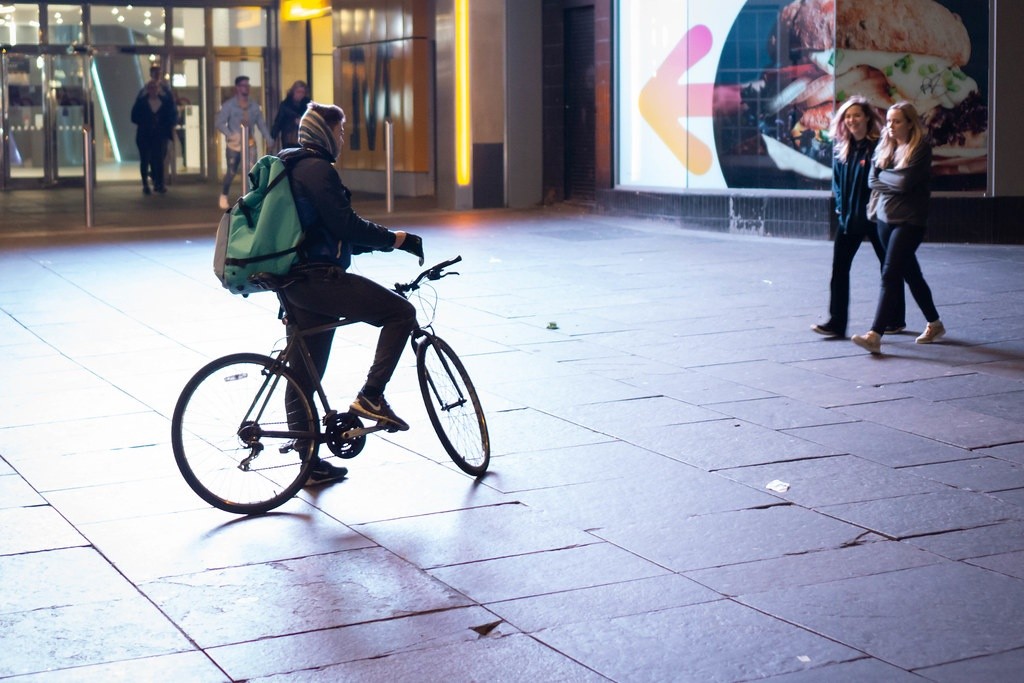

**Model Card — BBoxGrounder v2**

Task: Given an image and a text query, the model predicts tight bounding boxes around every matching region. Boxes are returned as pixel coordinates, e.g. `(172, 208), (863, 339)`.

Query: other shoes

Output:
(142, 185), (167, 195)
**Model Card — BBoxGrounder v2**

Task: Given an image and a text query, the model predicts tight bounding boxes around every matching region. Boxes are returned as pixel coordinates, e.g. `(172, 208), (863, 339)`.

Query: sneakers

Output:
(810, 317), (846, 339)
(300, 460), (348, 486)
(219, 194), (229, 209)
(851, 330), (881, 354)
(350, 392), (409, 432)
(916, 321), (946, 343)
(884, 323), (906, 334)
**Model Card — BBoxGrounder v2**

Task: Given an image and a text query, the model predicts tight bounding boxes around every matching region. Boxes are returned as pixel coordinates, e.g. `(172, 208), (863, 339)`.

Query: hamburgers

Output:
(742, 0), (991, 177)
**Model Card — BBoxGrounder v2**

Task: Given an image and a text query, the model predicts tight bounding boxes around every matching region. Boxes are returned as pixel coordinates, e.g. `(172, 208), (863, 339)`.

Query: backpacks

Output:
(213, 153), (333, 298)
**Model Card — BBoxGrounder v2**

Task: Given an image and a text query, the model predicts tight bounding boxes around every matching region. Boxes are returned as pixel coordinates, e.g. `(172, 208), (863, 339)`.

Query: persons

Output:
(130, 66), (180, 197)
(214, 76), (275, 209)
(270, 79), (312, 150)
(809, 96), (906, 337)
(849, 99), (947, 354)
(268, 102), (425, 489)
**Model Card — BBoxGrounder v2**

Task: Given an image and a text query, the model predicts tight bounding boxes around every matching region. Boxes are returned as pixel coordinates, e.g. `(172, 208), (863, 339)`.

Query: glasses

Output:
(237, 84), (251, 88)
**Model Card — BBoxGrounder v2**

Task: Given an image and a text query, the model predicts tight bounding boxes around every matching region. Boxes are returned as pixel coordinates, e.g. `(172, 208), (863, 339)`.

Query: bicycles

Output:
(170, 253), (492, 516)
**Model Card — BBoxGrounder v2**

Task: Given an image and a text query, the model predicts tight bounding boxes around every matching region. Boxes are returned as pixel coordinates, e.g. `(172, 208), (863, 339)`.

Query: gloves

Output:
(398, 233), (424, 267)
